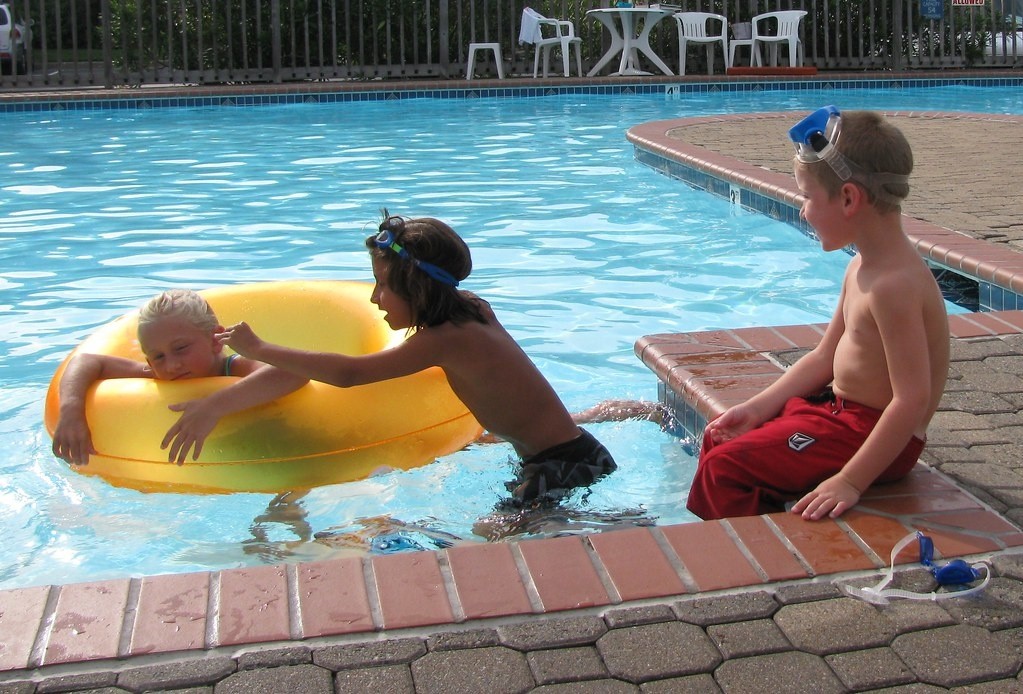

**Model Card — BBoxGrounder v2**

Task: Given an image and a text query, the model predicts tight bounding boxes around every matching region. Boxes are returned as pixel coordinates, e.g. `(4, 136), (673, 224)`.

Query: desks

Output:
(585, 8), (676, 76)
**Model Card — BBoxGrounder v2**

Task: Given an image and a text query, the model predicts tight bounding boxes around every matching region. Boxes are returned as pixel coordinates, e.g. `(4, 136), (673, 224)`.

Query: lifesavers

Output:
(45, 280), (483, 495)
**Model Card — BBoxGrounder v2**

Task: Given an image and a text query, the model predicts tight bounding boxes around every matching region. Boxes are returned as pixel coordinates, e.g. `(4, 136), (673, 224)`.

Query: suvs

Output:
(0, 3), (36, 77)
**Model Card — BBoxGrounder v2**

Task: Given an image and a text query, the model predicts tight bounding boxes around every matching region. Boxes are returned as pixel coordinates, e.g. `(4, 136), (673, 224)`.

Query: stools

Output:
(467, 43), (505, 79)
(728, 40), (762, 67)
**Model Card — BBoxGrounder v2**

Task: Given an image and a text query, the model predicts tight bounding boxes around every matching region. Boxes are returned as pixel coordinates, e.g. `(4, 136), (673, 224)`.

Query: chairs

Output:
(750, 10), (808, 68)
(672, 12), (729, 76)
(524, 7), (582, 78)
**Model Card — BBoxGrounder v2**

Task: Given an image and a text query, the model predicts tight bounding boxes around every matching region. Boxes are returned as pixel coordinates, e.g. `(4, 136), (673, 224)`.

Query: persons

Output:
(686, 105), (950, 523)
(50, 289), (311, 465)
(214, 208), (617, 501)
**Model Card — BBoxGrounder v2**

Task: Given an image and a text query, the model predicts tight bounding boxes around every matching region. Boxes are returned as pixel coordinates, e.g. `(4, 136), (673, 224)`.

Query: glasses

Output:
(919, 535), (978, 586)
(788, 105), (842, 164)
(374, 228), (394, 248)
